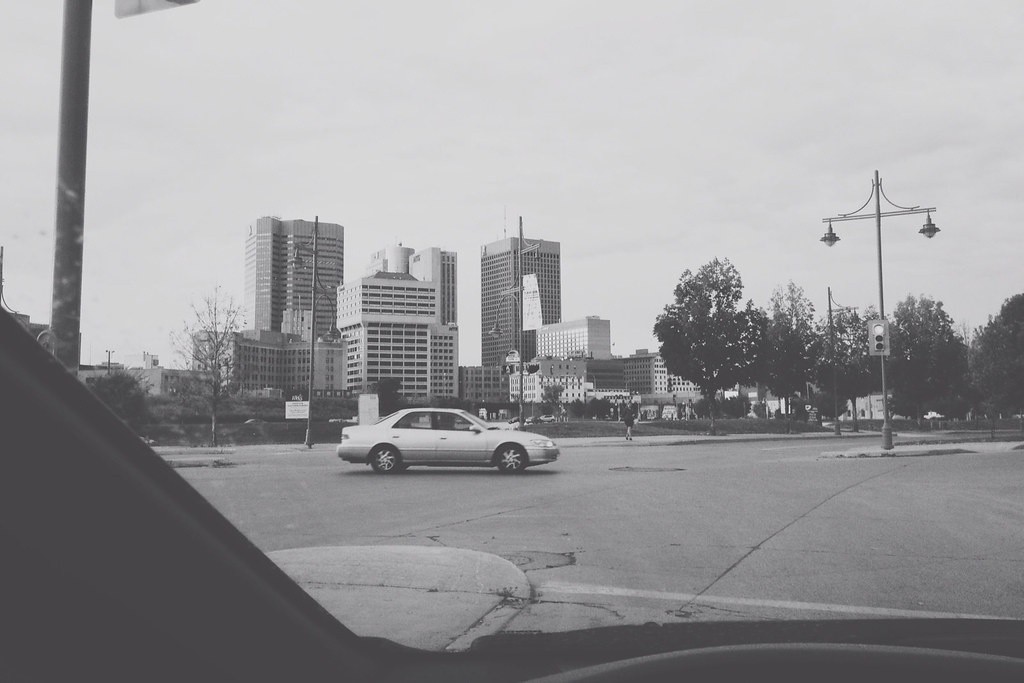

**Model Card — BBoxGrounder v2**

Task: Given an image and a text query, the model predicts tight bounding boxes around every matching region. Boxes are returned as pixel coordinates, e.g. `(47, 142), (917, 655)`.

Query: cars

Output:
(245, 419), (264, 423)
(508, 415), (526, 424)
(335, 406), (561, 476)
(525, 416), (541, 424)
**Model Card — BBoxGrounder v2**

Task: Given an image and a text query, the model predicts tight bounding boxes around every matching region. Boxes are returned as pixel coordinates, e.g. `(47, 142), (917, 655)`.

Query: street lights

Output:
(288, 216), (343, 449)
(826, 286), (859, 436)
(106, 350), (115, 375)
(487, 216), (541, 422)
(819, 169), (941, 450)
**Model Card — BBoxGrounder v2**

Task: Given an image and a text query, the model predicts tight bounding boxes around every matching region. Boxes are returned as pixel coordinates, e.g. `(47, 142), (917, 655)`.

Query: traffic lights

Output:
(867, 320), (890, 356)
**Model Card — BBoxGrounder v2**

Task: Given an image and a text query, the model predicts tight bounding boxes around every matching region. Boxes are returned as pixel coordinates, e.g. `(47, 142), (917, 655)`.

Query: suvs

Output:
(540, 414), (556, 423)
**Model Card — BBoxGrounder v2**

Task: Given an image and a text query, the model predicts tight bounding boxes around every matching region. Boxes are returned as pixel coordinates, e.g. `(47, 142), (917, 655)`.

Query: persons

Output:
(622, 403), (635, 440)
